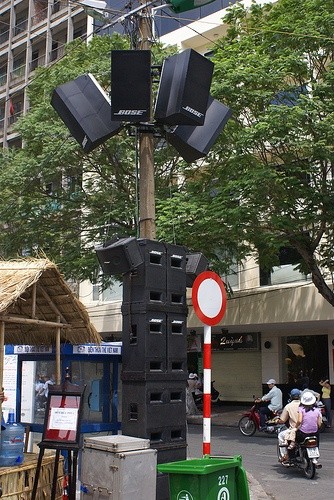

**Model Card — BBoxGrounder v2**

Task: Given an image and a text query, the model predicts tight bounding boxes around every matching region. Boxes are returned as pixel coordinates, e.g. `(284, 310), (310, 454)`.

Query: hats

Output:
(267, 378), (276, 384)
(303, 384), (309, 389)
(189, 373), (195, 378)
(301, 392), (316, 405)
(196, 381), (202, 386)
(291, 388), (301, 395)
(194, 374), (198, 377)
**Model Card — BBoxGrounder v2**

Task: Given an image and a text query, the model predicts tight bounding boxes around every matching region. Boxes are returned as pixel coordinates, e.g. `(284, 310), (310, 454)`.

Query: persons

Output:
(255, 376), (333, 461)
(185, 372), (204, 416)
(34, 374), (55, 414)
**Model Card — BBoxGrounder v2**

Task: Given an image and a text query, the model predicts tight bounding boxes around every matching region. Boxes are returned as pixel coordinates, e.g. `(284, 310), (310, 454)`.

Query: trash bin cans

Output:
(157, 454), (249, 500)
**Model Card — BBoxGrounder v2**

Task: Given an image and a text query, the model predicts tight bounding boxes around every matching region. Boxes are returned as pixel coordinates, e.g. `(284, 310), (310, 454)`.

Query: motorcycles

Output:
(274, 416), (322, 480)
(238, 395), (289, 438)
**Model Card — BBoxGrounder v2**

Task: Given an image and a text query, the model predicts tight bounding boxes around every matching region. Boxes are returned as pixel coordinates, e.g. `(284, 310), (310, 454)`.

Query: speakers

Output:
(153, 48), (214, 126)
(111, 50), (153, 123)
(50, 73), (126, 154)
(185, 252), (209, 288)
(168, 94), (231, 163)
(94, 236), (144, 274)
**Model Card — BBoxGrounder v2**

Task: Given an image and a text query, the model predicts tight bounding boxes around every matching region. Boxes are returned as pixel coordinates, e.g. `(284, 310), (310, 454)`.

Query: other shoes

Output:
(287, 443), (295, 450)
(279, 455), (290, 462)
(279, 442), (288, 447)
(289, 459), (295, 466)
(259, 426), (267, 430)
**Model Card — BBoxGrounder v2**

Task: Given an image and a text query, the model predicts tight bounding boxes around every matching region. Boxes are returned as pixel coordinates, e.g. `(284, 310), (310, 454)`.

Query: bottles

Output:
(0, 410), (25, 467)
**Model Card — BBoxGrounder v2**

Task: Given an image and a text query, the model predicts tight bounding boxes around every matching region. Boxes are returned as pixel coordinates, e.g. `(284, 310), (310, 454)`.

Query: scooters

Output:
(194, 380), (222, 406)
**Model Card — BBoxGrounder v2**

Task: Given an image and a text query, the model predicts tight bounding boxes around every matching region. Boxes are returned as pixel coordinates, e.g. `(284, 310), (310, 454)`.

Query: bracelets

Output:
(277, 420), (280, 424)
